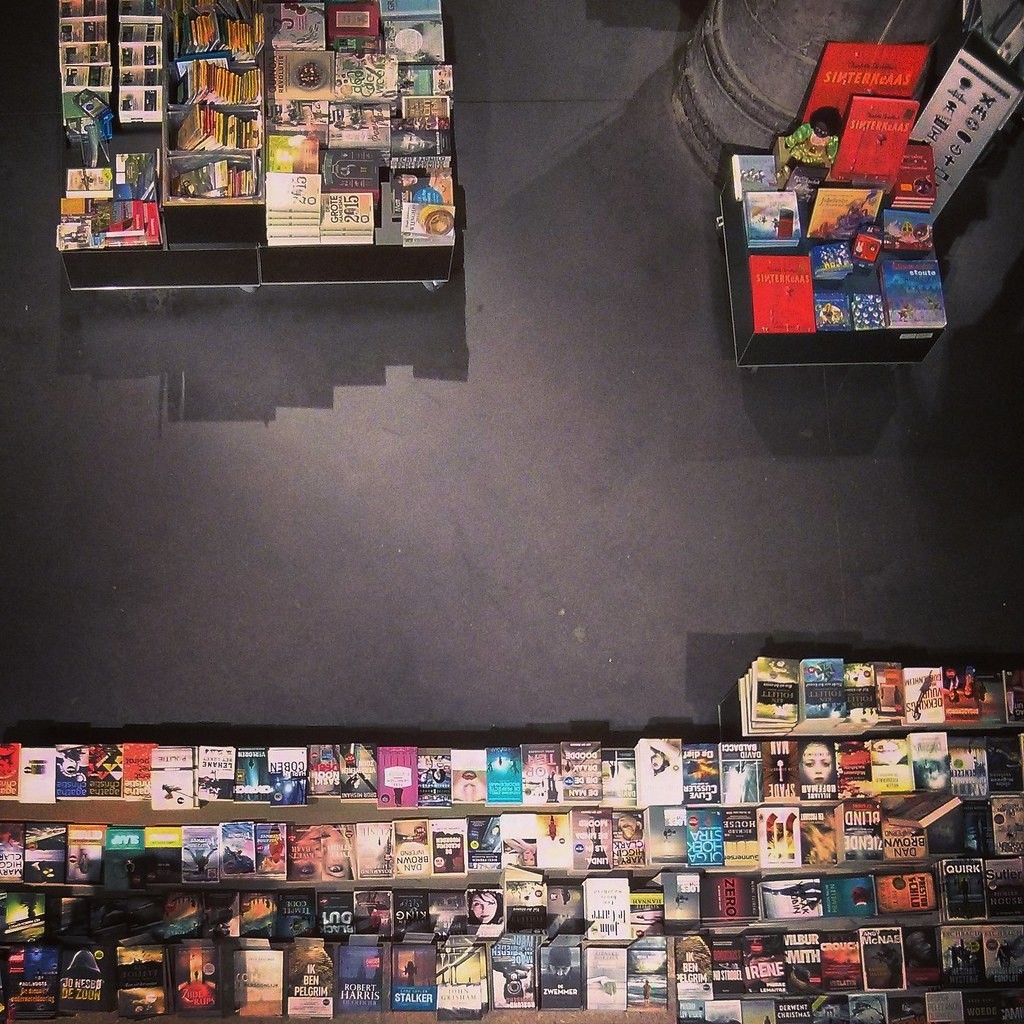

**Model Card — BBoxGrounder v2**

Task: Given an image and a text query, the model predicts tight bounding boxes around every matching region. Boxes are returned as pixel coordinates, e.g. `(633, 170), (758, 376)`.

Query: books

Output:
(56, 1), (457, 251)
(731, 96), (947, 334)
(0, 655), (1024, 1024)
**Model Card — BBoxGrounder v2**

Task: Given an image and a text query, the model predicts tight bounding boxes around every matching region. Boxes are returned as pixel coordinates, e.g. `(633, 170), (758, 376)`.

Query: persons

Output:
(880, 683), (885, 701)
(798, 741), (838, 799)
(392, 171), (436, 193)
(69, 844), (91, 881)
(391, 130), (439, 157)
(648, 739), (681, 776)
(905, 931), (936, 985)
(291, 825), (357, 882)
(467, 890), (503, 925)
(644, 979), (651, 1008)
(894, 686), (902, 711)
(961, 664), (981, 698)
(493, 961), (531, 1004)
(370, 908), (380, 932)
(270, 18), (294, 40)
(540, 947), (581, 993)
(617, 811), (644, 842)
(775, 107), (838, 182)
(995, 939), (1017, 970)
(943, 668), (960, 702)
(505, 837), (537, 868)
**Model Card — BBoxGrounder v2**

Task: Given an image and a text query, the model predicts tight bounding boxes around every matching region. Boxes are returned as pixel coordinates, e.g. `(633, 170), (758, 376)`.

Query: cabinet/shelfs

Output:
(55, 0), (467, 294)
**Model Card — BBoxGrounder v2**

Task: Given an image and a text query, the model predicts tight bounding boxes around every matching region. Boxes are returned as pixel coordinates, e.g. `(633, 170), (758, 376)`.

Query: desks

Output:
(715, 170), (949, 369)
